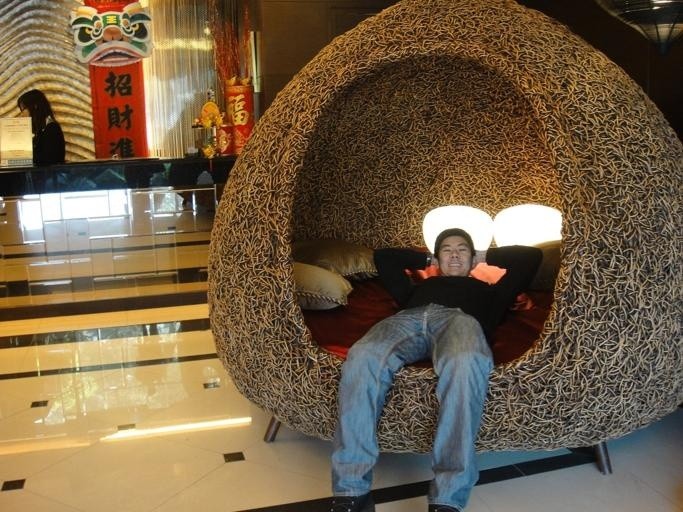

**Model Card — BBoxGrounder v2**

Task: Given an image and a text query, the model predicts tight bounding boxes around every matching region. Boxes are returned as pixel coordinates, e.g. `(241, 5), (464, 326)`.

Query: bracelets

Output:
(426, 251), (433, 270)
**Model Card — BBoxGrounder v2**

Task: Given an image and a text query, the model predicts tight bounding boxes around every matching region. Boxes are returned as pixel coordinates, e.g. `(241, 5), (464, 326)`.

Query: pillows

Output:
(521, 239), (564, 294)
(291, 237), (378, 310)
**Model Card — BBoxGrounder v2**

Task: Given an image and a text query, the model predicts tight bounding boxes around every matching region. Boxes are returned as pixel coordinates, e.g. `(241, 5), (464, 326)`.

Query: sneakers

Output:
(328, 488), (375, 512)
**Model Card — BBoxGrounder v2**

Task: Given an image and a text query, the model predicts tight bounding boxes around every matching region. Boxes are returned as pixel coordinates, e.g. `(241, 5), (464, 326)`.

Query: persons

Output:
(328, 229), (545, 512)
(16, 90), (63, 166)
